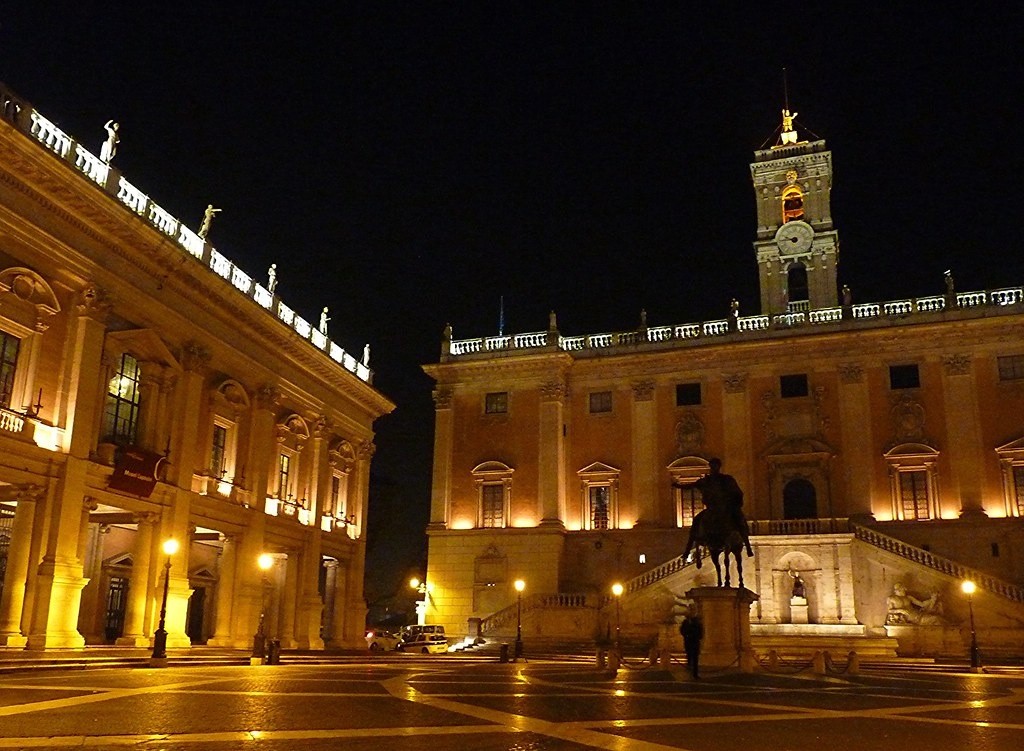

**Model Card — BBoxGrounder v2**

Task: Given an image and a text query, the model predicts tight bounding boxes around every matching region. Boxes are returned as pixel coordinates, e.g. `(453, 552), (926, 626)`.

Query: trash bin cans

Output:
(269, 638), (281, 665)
(500, 643), (510, 663)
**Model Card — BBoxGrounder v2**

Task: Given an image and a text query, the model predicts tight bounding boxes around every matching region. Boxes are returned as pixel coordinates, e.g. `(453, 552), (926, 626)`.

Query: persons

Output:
(548, 310), (559, 332)
(103, 118), (121, 165)
(364, 344), (370, 368)
(266, 261), (278, 296)
(319, 307), (331, 336)
(842, 284), (852, 306)
(640, 308), (647, 326)
(781, 108), (798, 133)
(787, 570), (805, 598)
(443, 321), (455, 345)
(196, 203), (222, 240)
(671, 458), (760, 560)
(729, 296), (740, 318)
(945, 271), (955, 295)
(885, 580), (953, 626)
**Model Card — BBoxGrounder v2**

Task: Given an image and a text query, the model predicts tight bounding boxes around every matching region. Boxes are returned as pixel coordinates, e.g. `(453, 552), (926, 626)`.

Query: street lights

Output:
(150, 539), (178, 667)
(250, 555), (273, 666)
(962, 581), (983, 674)
(513, 580), (527, 663)
(612, 584), (623, 641)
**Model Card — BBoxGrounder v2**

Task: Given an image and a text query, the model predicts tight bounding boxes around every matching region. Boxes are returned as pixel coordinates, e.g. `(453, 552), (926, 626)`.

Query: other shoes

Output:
(692, 673), (699, 679)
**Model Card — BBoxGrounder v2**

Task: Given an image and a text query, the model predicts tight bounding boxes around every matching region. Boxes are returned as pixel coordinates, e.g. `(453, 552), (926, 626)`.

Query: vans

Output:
(392, 624), (445, 642)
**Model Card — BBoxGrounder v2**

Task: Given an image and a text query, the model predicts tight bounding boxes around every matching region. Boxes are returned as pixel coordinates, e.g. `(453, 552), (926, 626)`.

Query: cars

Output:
(400, 633), (448, 654)
(364, 630), (402, 651)
